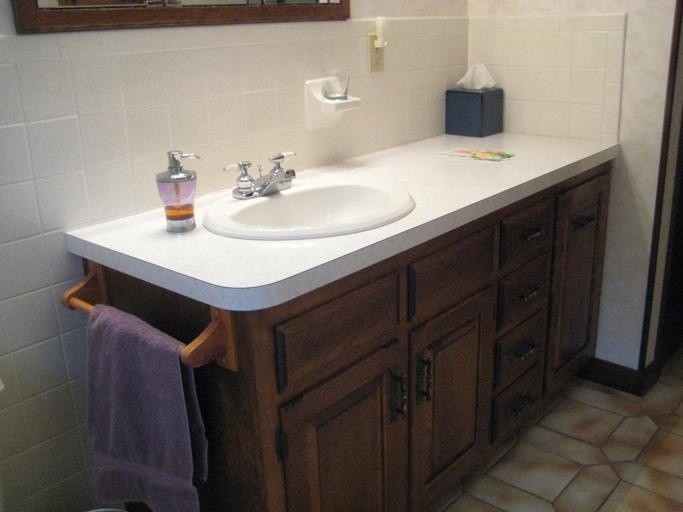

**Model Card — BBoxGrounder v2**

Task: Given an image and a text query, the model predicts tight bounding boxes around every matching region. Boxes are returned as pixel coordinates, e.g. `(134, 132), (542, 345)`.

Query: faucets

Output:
(258, 166), (297, 196)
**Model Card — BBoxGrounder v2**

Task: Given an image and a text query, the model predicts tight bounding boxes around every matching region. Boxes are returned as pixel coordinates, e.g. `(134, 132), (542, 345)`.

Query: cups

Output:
(321, 70), (349, 100)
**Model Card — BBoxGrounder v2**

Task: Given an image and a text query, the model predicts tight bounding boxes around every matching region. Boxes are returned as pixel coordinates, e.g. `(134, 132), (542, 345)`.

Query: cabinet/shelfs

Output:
(62, 195), (496, 512)
(493, 173), (555, 464)
(547, 150), (610, 402)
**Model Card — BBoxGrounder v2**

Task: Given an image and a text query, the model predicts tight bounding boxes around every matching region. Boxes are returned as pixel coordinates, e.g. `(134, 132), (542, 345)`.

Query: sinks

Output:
(203, 176), (418, 240)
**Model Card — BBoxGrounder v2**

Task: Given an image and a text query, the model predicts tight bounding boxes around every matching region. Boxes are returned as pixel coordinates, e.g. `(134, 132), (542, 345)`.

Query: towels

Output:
(87, 303), (207, 512)
